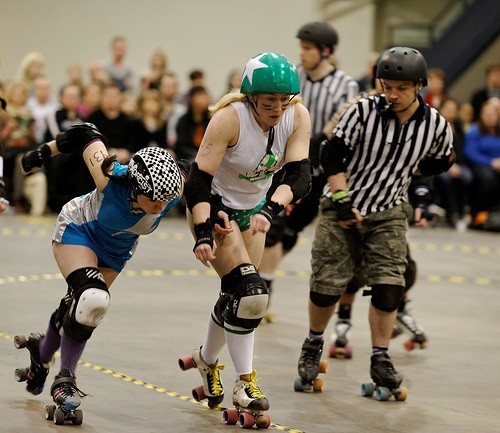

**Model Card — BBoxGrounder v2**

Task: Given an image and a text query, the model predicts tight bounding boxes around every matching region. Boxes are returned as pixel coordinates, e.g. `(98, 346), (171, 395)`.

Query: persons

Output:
(295, 46), (456, 402)
(177, 49), (312, 428)
(315, 79), (427, 358)
(13, 123), (185, 425)
(0, 34), (500, 231)
(244, 21), (359, 321)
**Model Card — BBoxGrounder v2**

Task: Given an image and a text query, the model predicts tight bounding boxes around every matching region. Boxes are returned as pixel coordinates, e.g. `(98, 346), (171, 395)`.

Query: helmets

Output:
(376, 46), (428, 87)
(128, 146), (182, 202)
(297, 22), (338, 46)
(239, 52), (301, 94)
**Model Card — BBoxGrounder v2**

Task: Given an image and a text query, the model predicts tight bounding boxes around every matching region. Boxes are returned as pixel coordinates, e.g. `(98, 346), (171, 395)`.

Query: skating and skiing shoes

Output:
(178, 345), (225, 404)
(360, 351), (408, 401)
(391, 312), (428, 350)
(14, 332), (55, 396)
(294, 337), (328, 392)
(223, 369), (271, 429)
(45, 368), (87, 425)
(328, 321), (352, 358)
